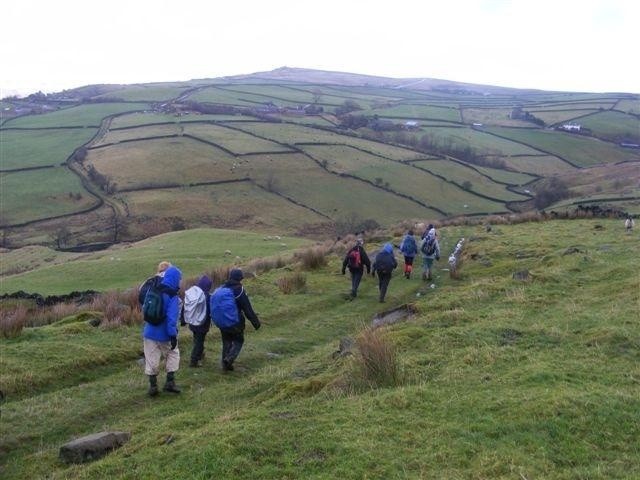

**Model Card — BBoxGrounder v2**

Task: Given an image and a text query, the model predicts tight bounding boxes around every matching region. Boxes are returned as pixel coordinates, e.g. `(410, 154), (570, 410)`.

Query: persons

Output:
(142, 267), (182, 395)
(419, 228), (440, 281)
(217, 268), (261, 373)
(180, 274), (215, 366)
(398, 230), (420, 279)
(623, 214), (635, 235)
(342, 236), (371, 298)
(137, 261), (174, 306)
(371, 242), (397, 302)
(421, 222), (437, 241)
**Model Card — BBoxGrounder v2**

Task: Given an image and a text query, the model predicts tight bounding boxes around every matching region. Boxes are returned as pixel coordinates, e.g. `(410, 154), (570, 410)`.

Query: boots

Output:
(404, 271), (433, 280)
(148, 353), (237, 396)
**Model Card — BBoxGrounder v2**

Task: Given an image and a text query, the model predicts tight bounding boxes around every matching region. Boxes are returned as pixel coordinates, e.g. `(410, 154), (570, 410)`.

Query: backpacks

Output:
(142, 287), (164, 326)
(376, 231), (437, 274)
(209, 287), (240, 329)
(348, 250), (361, 268)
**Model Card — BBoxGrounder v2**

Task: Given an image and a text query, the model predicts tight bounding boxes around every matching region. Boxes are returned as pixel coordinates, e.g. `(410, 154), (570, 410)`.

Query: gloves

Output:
(183, 284), (207, 326)
(170, 336), (177, 350)
